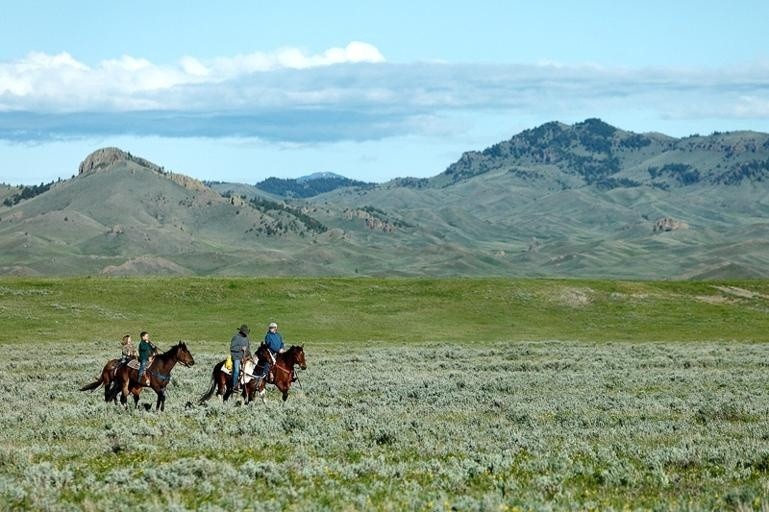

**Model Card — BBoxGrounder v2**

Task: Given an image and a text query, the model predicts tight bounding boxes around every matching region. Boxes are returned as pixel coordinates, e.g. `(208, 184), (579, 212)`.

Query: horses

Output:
(198, 341), (271, 405)
(78, 345), (158, 405)
(106, 340), (195, 412)
(241, 343), (307, 401)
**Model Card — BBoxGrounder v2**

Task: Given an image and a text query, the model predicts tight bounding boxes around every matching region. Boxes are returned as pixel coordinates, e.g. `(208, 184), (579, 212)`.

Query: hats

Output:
(269, 323), (277, 328)
(236, 324), (250, 335)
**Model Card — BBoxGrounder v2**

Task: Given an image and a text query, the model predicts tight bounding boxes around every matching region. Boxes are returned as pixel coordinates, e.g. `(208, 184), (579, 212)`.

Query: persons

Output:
(114, 334), (139, 387)
(259, 322), (286, 380)
(230, 323), (252, 393)
(135, 331), (158, 391)
